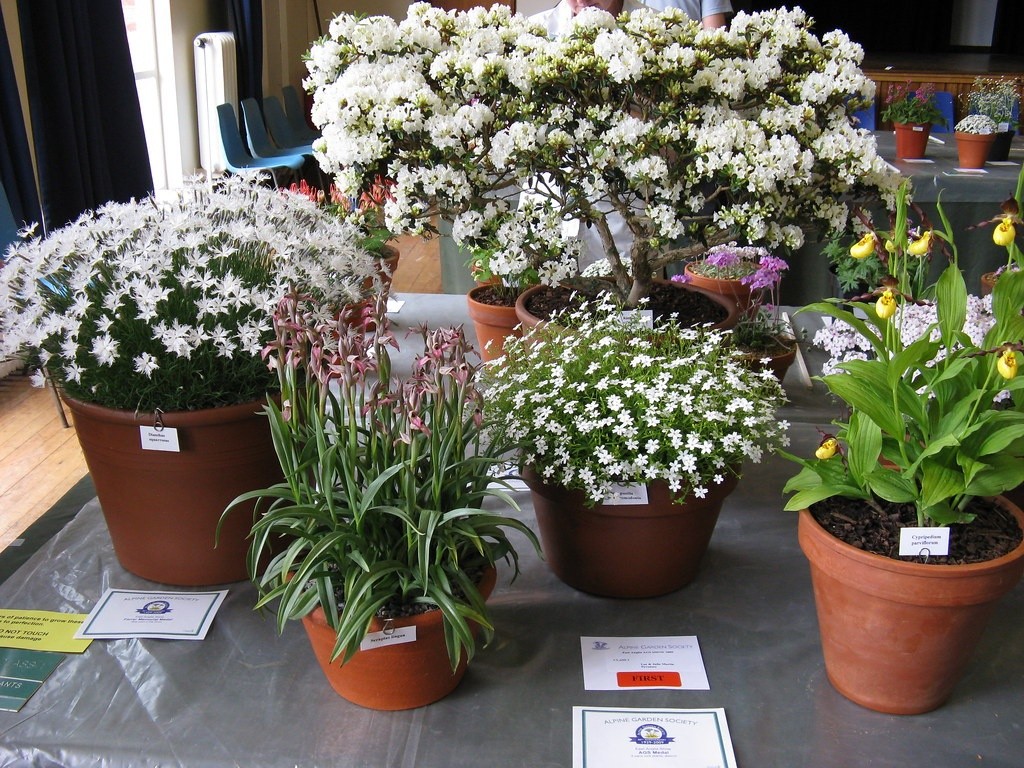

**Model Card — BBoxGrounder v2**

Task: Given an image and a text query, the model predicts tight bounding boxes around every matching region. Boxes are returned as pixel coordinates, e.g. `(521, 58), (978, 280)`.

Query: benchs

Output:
(217, 88), (323, 185)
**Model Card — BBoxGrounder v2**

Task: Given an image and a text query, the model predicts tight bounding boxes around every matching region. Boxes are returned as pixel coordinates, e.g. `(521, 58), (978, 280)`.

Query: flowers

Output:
(958, 76), (1021, 131)
(772, 178), (1024, 528)
(287, 179), (394, 260)
(302, 5), (903, 307)
(0, 167), (390, 412)
(215, 287), (545, 674)
(955, 114), (997, 136)
(708, 252), (805, 353)
(882, 81), (950, 131)
(475, 292), (791, 505)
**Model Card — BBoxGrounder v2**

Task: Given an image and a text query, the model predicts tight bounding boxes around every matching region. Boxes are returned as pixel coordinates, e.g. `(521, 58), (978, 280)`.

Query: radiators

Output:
(192, 30), (240, 180)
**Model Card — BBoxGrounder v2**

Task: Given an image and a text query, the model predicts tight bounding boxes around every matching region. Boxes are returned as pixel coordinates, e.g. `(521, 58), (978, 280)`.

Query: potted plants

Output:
(466, 259), (525, 364)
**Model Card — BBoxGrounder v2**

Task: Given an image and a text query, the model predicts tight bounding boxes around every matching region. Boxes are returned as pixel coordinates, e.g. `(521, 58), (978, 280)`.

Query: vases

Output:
(320, 303), (367, 377)
(681, 261), (767, 331)
(894, 122), (931, 158)
(987, 130), (1015, 162)
(954, 131), (997, 169)
(354, 247), (400, 329)
(798, 472), (1024, 716)
(518, 450), (740, 601)
(285, 559), (495, 712)
(727, 338), (796, 384)
(57, 378), (324, 588)
(515, 277), (739, 356)
